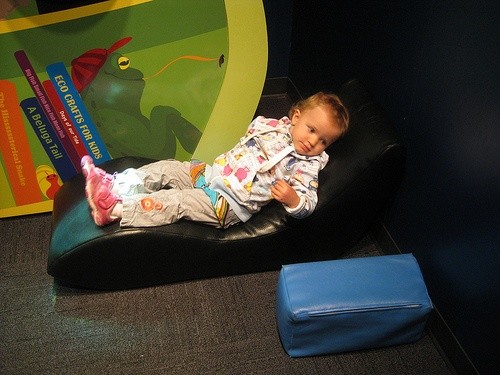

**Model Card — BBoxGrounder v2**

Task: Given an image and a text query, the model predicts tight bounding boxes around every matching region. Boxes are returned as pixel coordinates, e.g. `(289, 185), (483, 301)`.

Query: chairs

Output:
(47, 97), (408, 292)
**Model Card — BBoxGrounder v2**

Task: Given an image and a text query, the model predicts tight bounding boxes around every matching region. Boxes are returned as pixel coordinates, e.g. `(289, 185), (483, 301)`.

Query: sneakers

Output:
(81, 155), (113, 189)
(85, 172), (120, 226)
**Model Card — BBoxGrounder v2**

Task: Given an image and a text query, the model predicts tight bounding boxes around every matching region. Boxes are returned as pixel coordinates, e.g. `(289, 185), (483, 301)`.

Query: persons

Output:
(81, 92), (350, 228)
